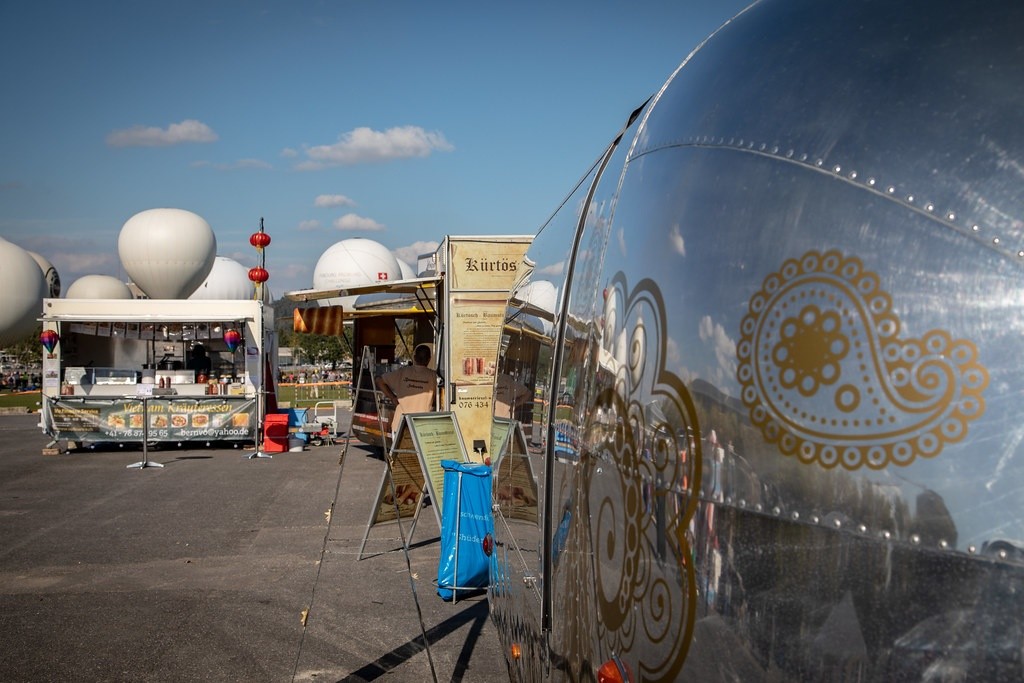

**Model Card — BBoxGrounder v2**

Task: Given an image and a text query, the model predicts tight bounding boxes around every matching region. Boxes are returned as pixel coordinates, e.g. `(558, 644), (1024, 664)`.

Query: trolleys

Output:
(310, 402), (337, 446)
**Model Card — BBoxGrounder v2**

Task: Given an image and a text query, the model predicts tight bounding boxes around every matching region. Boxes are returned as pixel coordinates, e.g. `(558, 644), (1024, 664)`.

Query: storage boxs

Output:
(263, 413), (289, 453)
(42, 448), (60, 456)
(288, 439), (304, 453)
(278, 402), (290, 409)
(244, 445), (260, 450)
(152, 388), (175, 395)
(277, 407), (309, 445)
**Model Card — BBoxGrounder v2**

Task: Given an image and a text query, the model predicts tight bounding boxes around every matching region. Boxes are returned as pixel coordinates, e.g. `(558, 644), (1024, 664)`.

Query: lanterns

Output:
(249, 217), (271, 300)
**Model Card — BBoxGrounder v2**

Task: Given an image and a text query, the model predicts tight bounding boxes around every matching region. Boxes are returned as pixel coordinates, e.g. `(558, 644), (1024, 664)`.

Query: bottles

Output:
(207, 373), (218, 385)
(219, 374), (233, 384)
(208, 382), (218, 395)
(159, 378), (164, 388)
(220, 384), (226, 395)
(60, 382), (67, 395)
(166, 377), (170, 388)
(197, 371), (207, 383)
(66, 385), (74, 395)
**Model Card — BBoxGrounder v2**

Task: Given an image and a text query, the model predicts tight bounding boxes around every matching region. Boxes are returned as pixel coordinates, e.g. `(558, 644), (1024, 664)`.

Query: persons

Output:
(186, 345), (211, 383)
(375, 345), (437, 443)
(277, 370), (353, 398)
(0, 371), (41, 392)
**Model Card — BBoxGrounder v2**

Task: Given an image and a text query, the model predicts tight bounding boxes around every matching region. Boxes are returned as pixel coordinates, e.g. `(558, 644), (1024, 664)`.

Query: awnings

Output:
(36, 315), (254, 325)
(276, 276), (444, 320)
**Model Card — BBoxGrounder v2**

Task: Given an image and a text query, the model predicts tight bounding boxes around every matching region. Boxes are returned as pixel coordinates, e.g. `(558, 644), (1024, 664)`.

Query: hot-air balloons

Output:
(223, 330), (241, 353)
(118, 208), (216, 299)
(314, 239), (419, 372)
(40, 330), (58, 358)
(0, 237), (61, 387)
(65, 274), (133, 299)
(187, 257), (274, 315)
